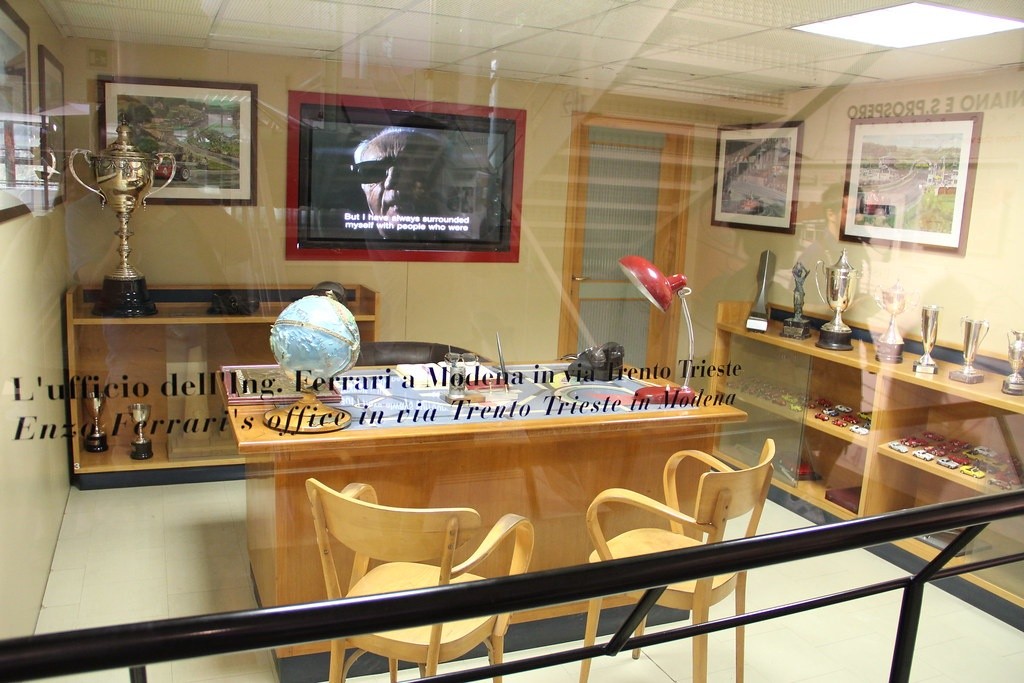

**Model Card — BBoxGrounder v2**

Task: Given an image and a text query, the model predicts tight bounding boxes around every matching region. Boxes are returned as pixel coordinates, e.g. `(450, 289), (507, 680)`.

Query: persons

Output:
(325, 126), (469, 240)
(794, 182), (874, 488)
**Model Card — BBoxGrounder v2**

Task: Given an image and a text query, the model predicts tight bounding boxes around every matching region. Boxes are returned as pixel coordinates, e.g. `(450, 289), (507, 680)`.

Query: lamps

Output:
(619, 256), (696, 405)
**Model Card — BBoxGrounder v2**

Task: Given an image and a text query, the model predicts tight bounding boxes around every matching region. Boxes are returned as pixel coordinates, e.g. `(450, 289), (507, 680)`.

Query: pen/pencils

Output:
(495, 330), (510, 394)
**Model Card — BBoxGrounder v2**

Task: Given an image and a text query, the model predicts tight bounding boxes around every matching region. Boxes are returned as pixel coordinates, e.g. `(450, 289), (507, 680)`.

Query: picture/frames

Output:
(839, 111), (984, 257)
(38, 44), (67, 210)
(0, 0), (35, 222)
(220, 365), (342, 405)
(711, 120), (805, 234)
(97, 75), (258, 206)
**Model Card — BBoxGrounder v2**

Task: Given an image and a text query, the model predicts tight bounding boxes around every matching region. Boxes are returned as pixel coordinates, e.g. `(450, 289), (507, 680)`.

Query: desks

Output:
(214, 362), (747, 683)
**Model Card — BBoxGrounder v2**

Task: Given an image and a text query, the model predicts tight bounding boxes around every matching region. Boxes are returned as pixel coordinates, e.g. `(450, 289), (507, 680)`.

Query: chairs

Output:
(305, 478), (535, 683)
(579, 438), (776, 683)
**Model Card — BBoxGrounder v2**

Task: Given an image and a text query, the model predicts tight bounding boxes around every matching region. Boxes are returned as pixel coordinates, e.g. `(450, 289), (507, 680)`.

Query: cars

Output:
(153, 161), (190, 181)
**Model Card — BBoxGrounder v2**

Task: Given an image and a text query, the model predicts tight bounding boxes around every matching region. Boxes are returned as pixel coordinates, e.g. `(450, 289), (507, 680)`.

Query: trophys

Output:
(127, 401), (155, 460)
(82, 391), (109, 452)
(780, 260), (813, 341)
(813, 247), (870, 352)
(948, 316), (988, 383)
(874, 277), (919, 364)
(1002, 328), (1024, 395)
(741, 250), (770, 333)
(912, 304), (944, 375)
(68, 114), (177, 317)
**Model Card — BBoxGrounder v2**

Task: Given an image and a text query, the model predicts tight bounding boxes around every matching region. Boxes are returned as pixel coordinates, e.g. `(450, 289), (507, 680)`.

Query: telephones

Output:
(567, 342), (625, 381)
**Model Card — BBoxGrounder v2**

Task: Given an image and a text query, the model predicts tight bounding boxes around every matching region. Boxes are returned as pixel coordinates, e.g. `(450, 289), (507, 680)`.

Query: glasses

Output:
(348, 160), (433, 183)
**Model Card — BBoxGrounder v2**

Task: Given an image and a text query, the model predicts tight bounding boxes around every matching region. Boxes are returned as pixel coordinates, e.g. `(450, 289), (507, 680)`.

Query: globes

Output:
(263, 281), (360, 434)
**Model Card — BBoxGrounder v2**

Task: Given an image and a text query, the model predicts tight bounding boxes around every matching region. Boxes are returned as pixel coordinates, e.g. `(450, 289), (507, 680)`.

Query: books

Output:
(395, 360), (504, 389)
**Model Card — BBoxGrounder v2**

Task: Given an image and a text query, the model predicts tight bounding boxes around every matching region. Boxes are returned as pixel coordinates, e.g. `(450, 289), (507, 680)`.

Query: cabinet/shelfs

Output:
(708, 300), (1024, 608)
(66, 284), (381, 490)
